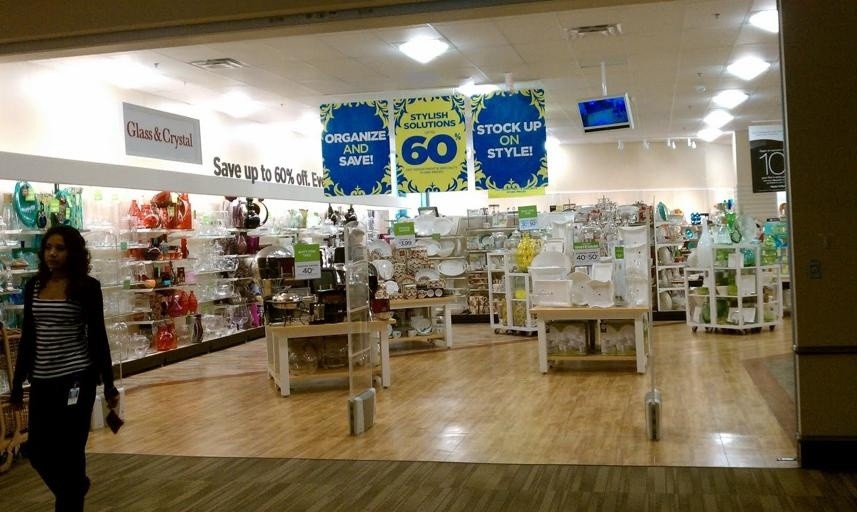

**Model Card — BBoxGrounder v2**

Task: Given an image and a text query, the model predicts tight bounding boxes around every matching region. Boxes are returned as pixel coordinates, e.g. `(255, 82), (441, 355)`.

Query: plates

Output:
(369, 239), (398, 298)
(567, 273), (616, 308)
(479, 234), (495, 248)
(413, 215), (469, 276)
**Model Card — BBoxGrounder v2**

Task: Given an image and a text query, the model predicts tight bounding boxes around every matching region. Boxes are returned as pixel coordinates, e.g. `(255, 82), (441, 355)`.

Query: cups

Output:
(408, 330), (417, 337)
(392, 331), (402, 338)
(404, 283), (428, 300)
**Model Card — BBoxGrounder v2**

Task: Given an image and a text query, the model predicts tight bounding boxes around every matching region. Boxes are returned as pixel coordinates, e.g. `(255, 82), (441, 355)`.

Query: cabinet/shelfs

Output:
(651, 191), (790, 336)
(386, 183), (538, 335)
(2, 185), (385, 405)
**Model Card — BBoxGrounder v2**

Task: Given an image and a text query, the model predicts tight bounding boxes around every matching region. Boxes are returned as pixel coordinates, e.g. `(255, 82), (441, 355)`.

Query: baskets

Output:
(0, 328), (21, 369)
(0, 394), (29, 436)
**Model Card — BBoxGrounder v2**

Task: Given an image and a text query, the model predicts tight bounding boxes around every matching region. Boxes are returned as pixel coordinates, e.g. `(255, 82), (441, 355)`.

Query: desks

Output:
(373, 289), (459, 353)
(527, 297), (653, 374)
(265, 315), (396, 398)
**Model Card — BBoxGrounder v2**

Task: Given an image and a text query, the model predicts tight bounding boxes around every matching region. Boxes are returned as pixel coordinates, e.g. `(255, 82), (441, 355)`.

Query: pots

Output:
(272, 291), (301, 310)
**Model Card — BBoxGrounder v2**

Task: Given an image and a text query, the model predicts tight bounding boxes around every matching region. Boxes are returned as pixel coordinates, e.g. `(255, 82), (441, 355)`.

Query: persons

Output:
(9, 224), (120, 512)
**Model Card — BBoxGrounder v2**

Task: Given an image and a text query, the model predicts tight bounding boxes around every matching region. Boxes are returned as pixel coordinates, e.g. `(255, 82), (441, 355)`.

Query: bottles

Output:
(151, 290), (203, 351)
(717, 223), (731, 244)
(128, 200), (163, 229)
(289, 352), (318, 375)
(224, 197), (260, 254)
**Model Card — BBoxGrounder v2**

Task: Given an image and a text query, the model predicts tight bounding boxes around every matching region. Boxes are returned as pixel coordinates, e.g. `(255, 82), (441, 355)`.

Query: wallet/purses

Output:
(105, 409), (124, 434)
